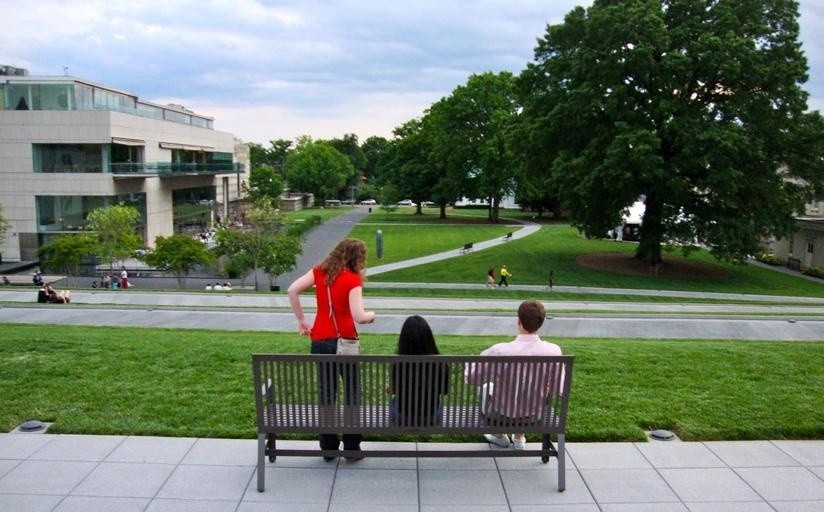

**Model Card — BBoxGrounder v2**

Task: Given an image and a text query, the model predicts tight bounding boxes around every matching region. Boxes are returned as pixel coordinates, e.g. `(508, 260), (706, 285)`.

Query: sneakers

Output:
(483, 434), (510, 447)
(511, 433), (526, 450)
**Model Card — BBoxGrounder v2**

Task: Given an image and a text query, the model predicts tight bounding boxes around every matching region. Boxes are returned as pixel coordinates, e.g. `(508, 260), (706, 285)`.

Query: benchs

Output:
(250, 352), (575, 493)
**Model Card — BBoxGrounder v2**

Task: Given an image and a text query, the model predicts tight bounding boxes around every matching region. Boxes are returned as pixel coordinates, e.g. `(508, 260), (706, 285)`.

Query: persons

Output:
(462, 299), (568, 452)
(37, 276), (43, 286)
(485, 265), (492, 287)
(547, 267), (556, 290)
(489, 268), (496, 290)
(497, 264), (511, 287)
(36, 283), (56, 303)
(286, 237), (377, 466)
(44, 286), (70, 304)
(32, 275), (38, 286)
(91, 265), (129, 289)
(387, 315), (455, 429)
(205, 282), (231, 291)
(2, 276), (10, 286)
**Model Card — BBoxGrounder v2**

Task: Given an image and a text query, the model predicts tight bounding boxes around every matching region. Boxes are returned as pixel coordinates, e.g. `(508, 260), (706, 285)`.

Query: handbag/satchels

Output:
(336, 337), (360, 355)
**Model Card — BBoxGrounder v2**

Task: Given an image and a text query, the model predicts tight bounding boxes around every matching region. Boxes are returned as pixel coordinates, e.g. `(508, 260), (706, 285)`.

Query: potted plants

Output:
(253, 229), (307, 293)
